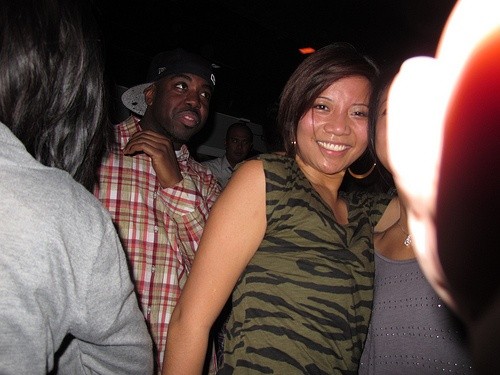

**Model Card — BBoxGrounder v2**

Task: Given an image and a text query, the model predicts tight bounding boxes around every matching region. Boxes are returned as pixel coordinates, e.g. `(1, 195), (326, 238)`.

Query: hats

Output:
(121, 53), (217, 116)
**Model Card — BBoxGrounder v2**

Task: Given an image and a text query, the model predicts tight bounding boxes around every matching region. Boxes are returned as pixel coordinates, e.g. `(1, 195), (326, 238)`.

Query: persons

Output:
(162, 40), (407, 375)
(351, 62), (474, 374)
(91, 49), (231, 375)
(0, 1), (159, 375)
(200, 121), (257, 192)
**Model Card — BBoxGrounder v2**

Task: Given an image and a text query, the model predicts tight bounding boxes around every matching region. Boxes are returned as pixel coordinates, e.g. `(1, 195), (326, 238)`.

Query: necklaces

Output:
(395, 221), (415, 248)
